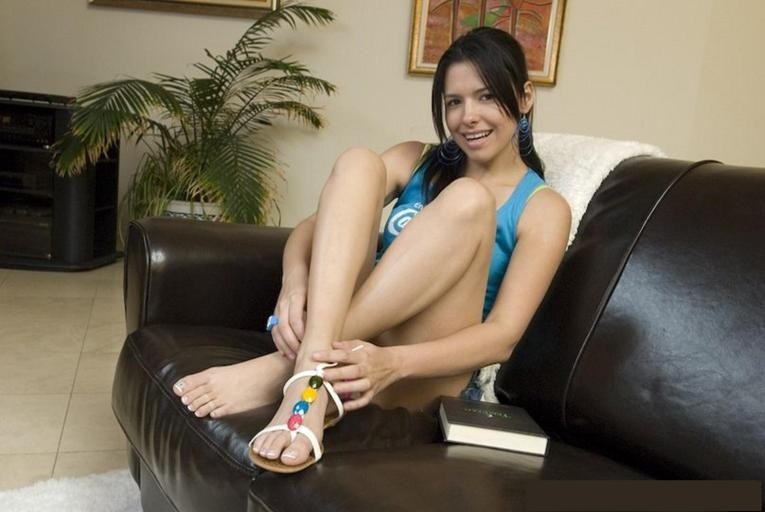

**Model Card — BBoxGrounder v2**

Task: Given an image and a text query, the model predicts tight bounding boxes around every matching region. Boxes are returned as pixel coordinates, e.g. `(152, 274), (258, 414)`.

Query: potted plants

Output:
(49, 3), (338, 223)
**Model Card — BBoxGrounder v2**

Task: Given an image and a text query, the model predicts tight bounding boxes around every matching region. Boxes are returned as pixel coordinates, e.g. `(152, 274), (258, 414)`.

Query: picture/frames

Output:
(407, 0), (568, 88)
(88, 0), (281, 19)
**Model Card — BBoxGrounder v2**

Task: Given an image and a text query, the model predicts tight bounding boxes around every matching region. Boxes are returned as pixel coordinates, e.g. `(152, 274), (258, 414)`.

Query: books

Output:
(437, 393), (550, 458)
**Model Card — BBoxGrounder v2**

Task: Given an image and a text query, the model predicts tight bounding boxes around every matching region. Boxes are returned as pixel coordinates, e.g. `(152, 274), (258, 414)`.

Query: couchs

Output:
(110, 158), (765, 512)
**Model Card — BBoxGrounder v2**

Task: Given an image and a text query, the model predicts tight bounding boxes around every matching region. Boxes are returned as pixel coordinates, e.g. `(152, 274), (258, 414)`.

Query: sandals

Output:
(247, 360), (345, 475)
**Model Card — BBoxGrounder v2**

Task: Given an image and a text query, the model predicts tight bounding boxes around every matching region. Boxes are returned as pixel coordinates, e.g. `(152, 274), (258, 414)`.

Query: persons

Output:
(169, 25), (574, 476)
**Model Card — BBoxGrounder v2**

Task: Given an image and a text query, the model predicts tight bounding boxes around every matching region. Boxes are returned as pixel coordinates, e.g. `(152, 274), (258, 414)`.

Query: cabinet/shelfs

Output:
(0, 89), (134, 271)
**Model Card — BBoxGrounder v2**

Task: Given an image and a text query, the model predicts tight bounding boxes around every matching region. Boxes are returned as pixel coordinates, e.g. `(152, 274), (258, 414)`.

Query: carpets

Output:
(0, 468), (142, 512)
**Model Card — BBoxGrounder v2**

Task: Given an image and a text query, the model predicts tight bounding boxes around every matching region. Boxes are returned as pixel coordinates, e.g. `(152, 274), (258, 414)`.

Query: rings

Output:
(264, 316), (278, 329)
